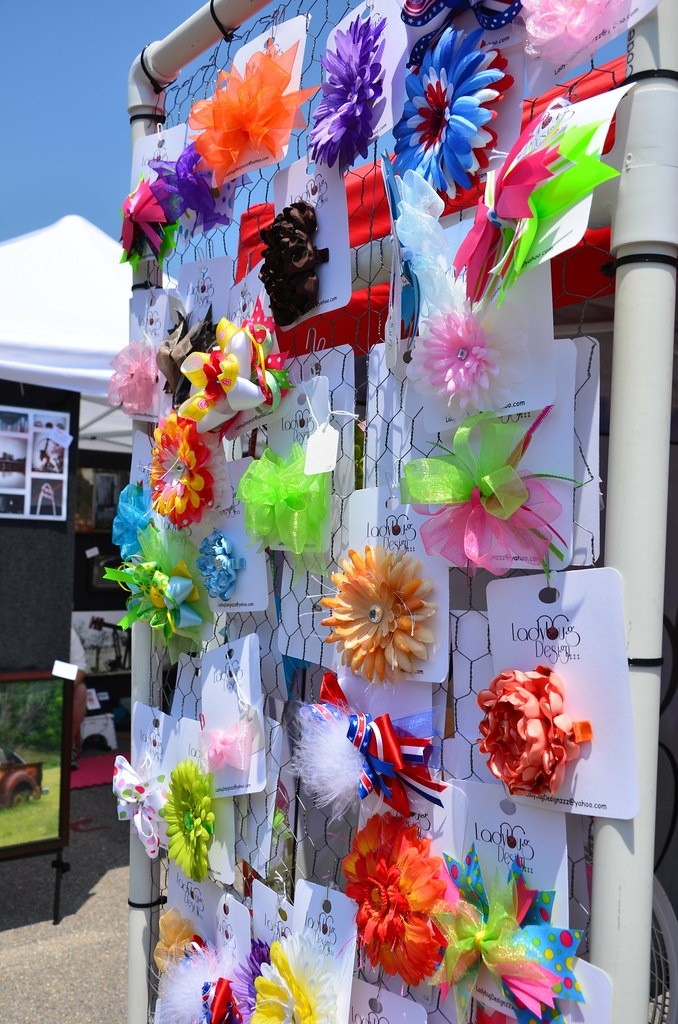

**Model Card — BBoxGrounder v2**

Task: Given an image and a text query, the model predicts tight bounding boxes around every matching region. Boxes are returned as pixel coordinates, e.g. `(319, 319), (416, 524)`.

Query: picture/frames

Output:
(0, 672), (75, 862)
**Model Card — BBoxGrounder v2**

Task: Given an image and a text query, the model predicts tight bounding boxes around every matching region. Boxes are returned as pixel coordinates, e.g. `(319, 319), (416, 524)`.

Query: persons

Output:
(70, 627), (87, 769)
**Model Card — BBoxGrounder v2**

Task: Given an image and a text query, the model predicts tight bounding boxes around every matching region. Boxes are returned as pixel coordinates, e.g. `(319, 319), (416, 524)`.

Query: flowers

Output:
(176, 298), (299, 447)
(153, 907), (196, 973)
(339, 813), (464, 990)
(149, 409), (215, 530)
(144, 144), (251, 239)
(186, 33), (322, 191)
(405, 299), (508, 412)
(320, 545), (437, 684)
(112, 481), (154, 558)
(195, 530), (247, 602)
(229, 937), (270, 1024)
(160, 756), (220, 883)
(250, 932), (337, 1024)
(236, 443), (329, 593)
(476, 664), (594, 798)
(107, 331), (161, 413)
(393, 25), (514, 202)
(257, 200), (330, 328)
(307, 13), (390, 167)
(103, 519), (218, 665)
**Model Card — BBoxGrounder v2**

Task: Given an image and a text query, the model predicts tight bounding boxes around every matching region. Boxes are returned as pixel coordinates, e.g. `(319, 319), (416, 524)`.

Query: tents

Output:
(0, 213), (180, 454)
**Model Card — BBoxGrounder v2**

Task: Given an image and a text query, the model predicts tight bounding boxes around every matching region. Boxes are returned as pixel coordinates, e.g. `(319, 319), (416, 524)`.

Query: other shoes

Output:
(70, 748), (78, 768)
